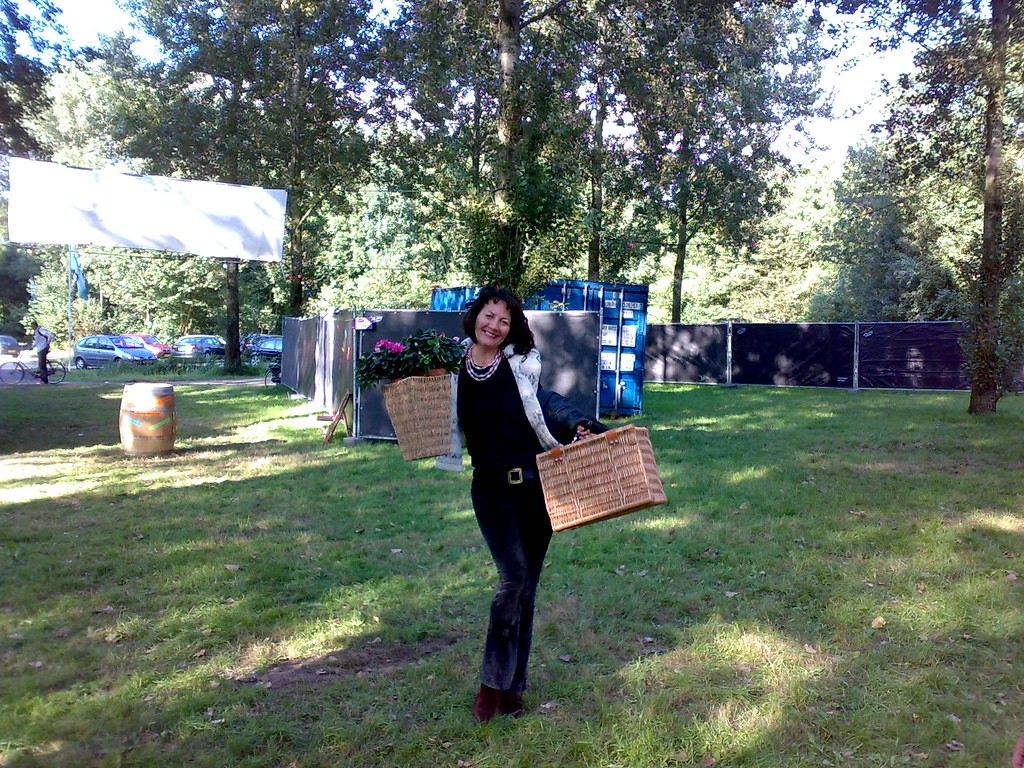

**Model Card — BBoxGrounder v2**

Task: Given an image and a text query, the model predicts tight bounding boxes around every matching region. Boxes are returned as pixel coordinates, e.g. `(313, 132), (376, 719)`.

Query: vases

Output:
(392, 368), (448, 384)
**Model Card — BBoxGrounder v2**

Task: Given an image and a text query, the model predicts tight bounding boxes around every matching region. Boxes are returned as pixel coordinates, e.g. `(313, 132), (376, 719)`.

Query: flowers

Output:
(353, 328), (467, 391)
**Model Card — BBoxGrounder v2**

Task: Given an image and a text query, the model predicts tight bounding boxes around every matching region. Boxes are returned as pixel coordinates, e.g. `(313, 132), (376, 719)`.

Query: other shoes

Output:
(475, 684), (501, 723)
(499, 691), (522, 718)
(36, 382), (50, 385)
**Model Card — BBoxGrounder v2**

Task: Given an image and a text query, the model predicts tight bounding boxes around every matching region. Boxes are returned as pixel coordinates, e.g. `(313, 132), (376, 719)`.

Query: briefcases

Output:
(535, 424), (668, 532)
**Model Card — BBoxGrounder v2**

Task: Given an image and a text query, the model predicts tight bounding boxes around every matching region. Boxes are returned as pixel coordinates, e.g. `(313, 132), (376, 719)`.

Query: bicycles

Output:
(263, 356), (282, 391)
(0, 355), (66, 386)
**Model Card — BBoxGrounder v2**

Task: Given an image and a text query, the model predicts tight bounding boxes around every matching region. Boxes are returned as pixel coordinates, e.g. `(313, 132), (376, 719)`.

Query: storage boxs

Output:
(430, 279), (648, 422)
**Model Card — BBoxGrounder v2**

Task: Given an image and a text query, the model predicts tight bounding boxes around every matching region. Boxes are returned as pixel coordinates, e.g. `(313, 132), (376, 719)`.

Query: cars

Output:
(239, 333), (282, 356)
(246, 338), (283, 367)
(172, 334), (226, 364)
(0, 335), (22, 359)
(73, 335), (158, 372)
(114, 332), (173, 359)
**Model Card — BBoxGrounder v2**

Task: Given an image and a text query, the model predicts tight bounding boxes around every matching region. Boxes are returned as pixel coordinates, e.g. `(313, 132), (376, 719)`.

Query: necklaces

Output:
(464, 344), (502, 383)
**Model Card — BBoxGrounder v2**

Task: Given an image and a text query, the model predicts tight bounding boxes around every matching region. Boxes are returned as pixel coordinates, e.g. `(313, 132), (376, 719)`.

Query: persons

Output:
(452, 283), (590, 723)
(30, 321), (53, 385)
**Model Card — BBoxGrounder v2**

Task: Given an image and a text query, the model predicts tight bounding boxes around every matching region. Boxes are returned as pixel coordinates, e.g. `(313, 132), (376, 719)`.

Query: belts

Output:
(476, 465), (538, 485)
